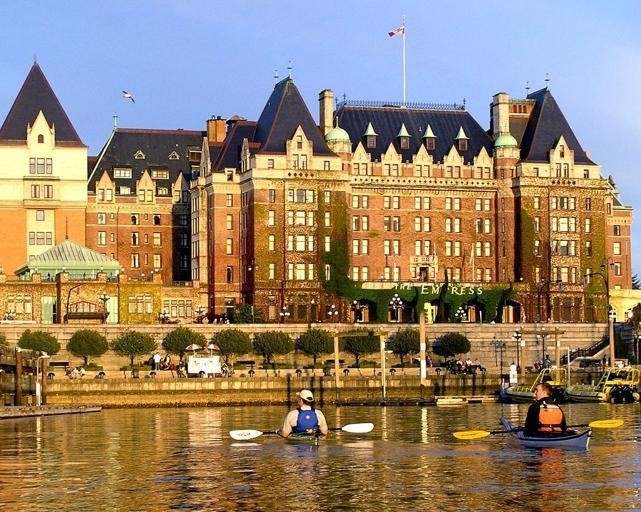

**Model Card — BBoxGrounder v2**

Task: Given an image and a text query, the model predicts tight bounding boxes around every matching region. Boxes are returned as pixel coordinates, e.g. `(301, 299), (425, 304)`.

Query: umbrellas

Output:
(185, 342), (203, 355)
(204, 342), (220, 356)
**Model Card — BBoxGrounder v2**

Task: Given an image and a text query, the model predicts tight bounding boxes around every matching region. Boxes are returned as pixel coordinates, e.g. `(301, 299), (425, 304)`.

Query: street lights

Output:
(354, 300), (358, 323)
(66, 282), (89, 325)
(453, 307), (467, 322)
(390, 293), (403, 323)
(278, 307), (290, 324)
(537, 280), (561, 325)
(98, 291), (109, 325)
(491, 327), (551, 378)
(327, 304), (338, 323)
(581, 272), (611, 324)
(34, 352), (52, 406)
(159, 309), (169, 323)
(194, 304), (205, 323)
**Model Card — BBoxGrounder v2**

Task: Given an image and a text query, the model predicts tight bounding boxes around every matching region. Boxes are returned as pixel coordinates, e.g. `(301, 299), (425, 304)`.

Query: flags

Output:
(389, 25), (405, 38)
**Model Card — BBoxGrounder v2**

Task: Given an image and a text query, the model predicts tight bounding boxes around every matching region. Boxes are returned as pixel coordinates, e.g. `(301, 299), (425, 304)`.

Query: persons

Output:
(177, 360), (187, 378)
(150, 349), (175, 371)
(603, 353), (608, 365)
(510, 361), (519, 387)
(526, 383), (569, 436)
(448, 357), (488, 375)
(65, 365), (86, 378)
(275, 390), (329, 437)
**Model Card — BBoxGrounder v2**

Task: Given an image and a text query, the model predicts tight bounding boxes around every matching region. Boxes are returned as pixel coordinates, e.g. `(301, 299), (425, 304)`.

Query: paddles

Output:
(454, 419), (624, 439)
(229, 423), (375, 440)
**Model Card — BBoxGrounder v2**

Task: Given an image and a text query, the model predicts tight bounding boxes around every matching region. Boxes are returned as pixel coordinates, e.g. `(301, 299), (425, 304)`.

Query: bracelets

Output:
(277, 429), (281, 434)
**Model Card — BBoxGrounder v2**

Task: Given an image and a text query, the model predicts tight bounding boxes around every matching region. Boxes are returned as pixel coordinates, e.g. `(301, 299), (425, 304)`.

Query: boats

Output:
(566, 366), (641, 405)
(500, 416), (593, 450)
(282, 431), (320, 449)
(507, 363), (568, 405)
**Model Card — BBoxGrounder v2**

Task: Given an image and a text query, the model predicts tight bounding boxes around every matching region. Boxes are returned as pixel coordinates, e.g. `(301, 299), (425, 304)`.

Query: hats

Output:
(296, 390), (315, 402)
